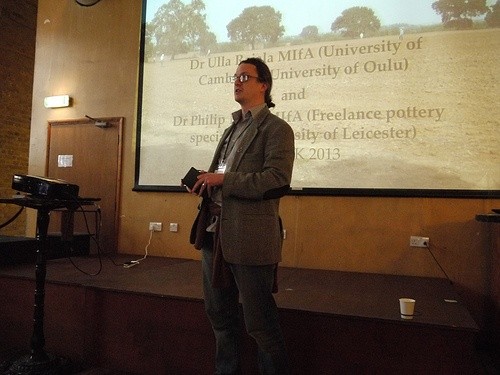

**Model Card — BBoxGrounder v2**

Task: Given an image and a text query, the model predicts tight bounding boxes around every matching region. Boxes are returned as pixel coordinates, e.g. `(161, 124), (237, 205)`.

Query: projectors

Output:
(10, 173), (78, 201)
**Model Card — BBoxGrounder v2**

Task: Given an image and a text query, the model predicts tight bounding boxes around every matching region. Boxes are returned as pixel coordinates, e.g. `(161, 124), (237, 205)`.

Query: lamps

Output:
(43, 94), (70, 108)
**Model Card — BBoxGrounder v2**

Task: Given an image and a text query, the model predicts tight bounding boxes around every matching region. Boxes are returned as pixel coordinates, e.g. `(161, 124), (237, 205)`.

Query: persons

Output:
(180, 58), (295, 375)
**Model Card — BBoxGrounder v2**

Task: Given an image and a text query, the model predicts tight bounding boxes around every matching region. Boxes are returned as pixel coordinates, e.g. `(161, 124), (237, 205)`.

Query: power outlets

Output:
(410, 236), (429, 248)
(149, 222), (162, 231)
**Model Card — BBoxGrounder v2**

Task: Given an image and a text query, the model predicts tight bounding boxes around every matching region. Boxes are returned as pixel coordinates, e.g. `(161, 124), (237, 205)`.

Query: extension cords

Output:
(124, 261), (139, 268)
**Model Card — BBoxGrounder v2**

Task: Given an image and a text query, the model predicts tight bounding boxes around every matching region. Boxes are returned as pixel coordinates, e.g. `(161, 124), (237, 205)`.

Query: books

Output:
(181, 167), (214, 198)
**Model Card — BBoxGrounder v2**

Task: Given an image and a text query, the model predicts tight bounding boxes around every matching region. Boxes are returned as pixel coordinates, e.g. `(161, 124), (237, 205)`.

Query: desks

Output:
(0, 196), (102, 375)
(51, 208), (96, 257)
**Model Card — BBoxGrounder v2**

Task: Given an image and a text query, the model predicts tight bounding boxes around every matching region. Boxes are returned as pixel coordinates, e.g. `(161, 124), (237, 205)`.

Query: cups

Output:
(399, 299), (416, 320)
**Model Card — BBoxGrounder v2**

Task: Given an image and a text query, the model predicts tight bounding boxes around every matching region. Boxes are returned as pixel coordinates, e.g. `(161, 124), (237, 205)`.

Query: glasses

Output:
(232, 74), (263, 84)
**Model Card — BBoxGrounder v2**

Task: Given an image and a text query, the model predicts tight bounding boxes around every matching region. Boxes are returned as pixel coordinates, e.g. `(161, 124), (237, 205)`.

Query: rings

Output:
(203, 183), (206, 187)
